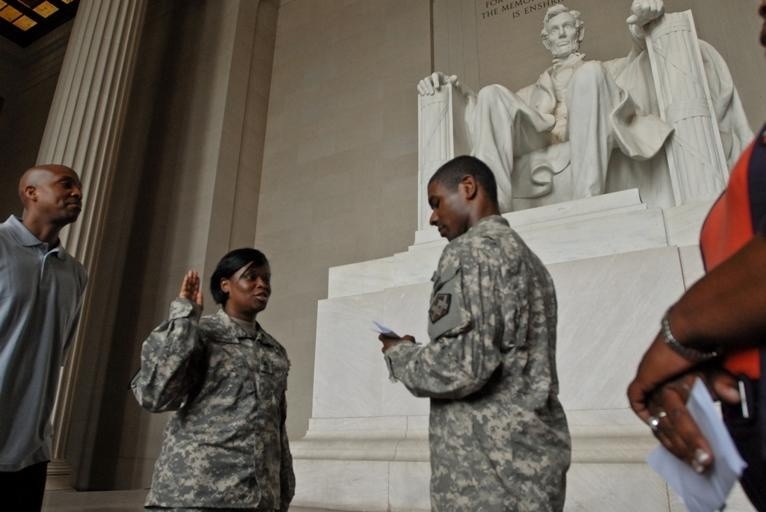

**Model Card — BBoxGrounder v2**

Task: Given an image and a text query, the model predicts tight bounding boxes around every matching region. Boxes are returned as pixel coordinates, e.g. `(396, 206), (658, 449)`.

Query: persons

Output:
(379, 156), (571, 512)
(130, 248), (296, 512)
(416, 0), (666, 213)
(627, 0), (766, 512)
(0, 164), (88, 512)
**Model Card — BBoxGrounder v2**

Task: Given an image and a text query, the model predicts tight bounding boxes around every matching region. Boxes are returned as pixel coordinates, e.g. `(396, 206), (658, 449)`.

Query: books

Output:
(645, 377), (748, 512)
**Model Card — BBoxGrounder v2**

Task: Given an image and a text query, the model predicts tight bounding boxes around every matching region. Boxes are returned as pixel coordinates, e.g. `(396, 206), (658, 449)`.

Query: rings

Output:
(648, 410), (667, 431)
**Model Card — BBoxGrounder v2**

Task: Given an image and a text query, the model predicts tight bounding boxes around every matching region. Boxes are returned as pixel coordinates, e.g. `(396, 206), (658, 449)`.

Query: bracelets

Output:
(662, 314), (718, 364)
